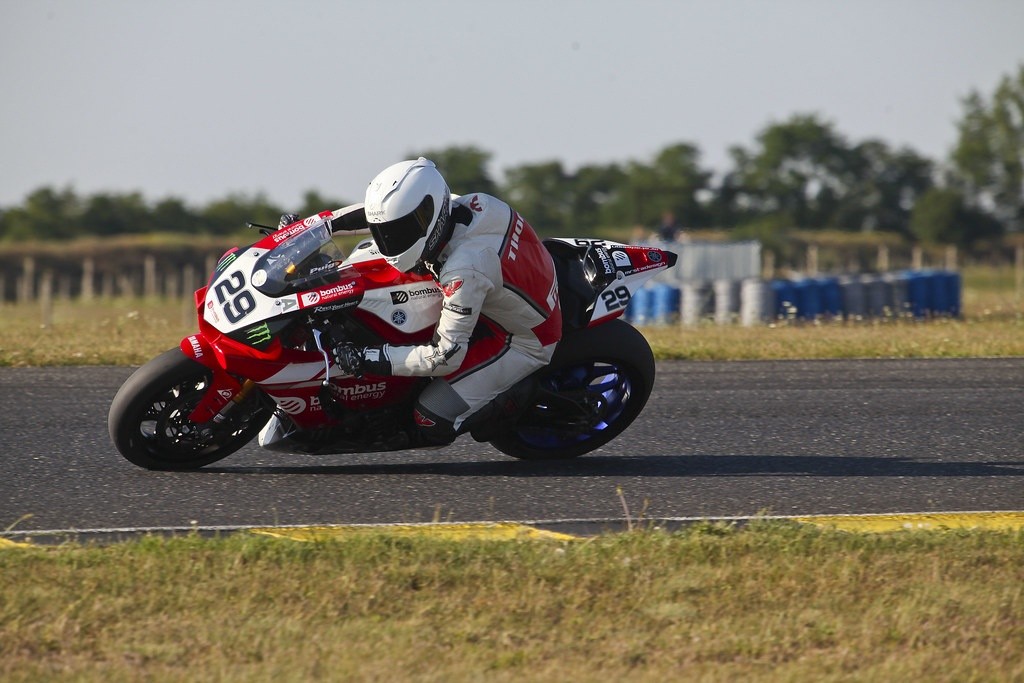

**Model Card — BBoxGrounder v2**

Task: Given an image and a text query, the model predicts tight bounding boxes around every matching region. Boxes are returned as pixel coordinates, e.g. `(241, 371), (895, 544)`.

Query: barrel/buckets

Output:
(622, 271), (959, 328)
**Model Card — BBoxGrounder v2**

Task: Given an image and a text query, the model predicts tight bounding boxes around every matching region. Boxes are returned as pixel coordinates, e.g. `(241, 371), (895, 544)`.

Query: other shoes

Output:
(470, 393), (530, 443)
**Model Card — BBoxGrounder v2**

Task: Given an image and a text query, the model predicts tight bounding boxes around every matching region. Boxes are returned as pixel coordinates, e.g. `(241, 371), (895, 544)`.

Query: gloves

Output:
(333, 342), (393, 378)
(278, 214), (299, 232)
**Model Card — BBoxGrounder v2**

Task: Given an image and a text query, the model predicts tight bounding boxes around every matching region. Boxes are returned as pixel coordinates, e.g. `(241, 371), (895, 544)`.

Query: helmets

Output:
(364, 157), (452, 273)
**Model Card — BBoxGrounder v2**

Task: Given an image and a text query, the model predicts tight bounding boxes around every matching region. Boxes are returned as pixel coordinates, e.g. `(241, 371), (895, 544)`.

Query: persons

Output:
(276, 155), (562, 446)
